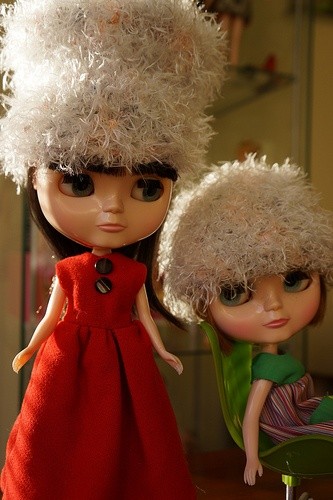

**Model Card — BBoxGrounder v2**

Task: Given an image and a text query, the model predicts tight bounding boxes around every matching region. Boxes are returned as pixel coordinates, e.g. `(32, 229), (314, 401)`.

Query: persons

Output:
(0, 1), (227, 500)
(156, 161), (332, 486)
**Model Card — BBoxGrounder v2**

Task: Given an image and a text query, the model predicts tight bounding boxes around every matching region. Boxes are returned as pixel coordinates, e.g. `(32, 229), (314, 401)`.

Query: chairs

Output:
(199, 321), (333, 500)
